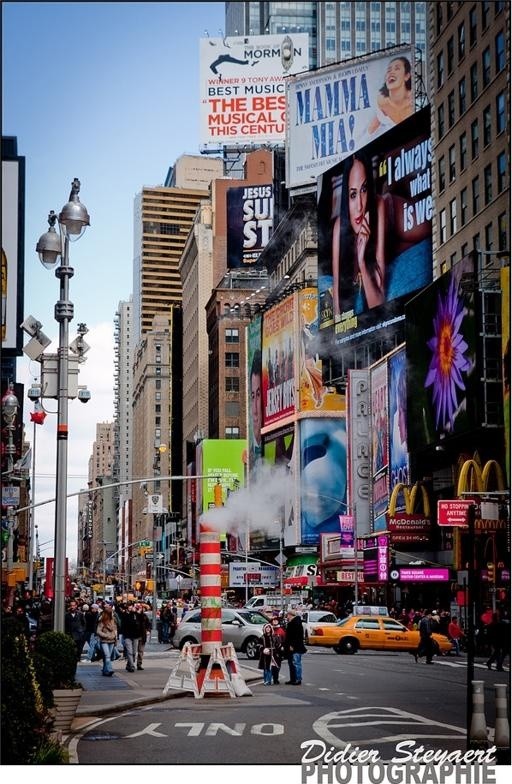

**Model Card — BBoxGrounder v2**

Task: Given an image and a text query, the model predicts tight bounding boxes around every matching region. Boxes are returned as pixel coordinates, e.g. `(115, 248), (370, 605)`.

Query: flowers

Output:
(422, 268), (474, 434)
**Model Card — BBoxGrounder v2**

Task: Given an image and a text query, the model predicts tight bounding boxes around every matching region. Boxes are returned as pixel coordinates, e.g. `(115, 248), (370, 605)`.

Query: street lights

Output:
(37, 179), (91, 634)
(308, 490), (359, 608)
(150, 441), (167, 643)
(4, 386), (21, 572)
(170, 543), (285, 613)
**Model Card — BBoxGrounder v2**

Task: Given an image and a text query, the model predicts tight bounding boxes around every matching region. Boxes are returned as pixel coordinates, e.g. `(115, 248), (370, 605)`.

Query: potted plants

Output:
(30, 629), (86, 736)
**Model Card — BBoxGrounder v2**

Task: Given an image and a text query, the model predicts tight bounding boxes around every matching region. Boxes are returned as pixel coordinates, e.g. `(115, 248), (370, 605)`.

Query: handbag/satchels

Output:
(90, 640), (104, 662)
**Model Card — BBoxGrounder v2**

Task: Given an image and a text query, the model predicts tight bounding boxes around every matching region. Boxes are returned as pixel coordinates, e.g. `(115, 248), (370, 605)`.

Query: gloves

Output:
(263, 648), (270, 657)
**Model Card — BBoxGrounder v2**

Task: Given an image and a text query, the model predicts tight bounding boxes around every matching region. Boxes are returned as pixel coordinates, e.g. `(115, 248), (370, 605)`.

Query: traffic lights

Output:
(134, 581), (145, 591)
(6, 571), (17, 588)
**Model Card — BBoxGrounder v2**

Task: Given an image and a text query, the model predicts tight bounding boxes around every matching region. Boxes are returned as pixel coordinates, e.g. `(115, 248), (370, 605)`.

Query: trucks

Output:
(243, 595), (304, 612)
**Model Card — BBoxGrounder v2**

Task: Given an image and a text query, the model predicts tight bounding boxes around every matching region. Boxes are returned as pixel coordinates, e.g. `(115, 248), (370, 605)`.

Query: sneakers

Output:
(263, 680), (302, 686)
(484, 661), (505, 672)
(412, 650), (434, 666)
(101, 665), (145, 677)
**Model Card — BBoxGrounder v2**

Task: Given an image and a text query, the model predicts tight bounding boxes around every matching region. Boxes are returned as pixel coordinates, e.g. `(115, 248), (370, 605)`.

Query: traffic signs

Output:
(144, 551), (165, 560)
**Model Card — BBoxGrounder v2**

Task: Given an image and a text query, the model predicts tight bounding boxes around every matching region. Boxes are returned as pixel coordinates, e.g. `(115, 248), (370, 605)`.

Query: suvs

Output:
(172, 608), (280, 660)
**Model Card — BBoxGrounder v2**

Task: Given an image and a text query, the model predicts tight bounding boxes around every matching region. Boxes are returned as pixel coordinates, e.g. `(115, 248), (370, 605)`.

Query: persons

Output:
(258, 624), (284, 686)
(284, 609), (307, 685)
(0, 596), (243, 676)
(389, 608), (509, 672)
(300, 313), (334, 408)
(269, 616), (286, 685)
(303, 597), (361, 621)
(249, 349), (262, 488)
(368, 56), (412, 134)
(332, 149), (431, 315)
(209, 38), (259, 81)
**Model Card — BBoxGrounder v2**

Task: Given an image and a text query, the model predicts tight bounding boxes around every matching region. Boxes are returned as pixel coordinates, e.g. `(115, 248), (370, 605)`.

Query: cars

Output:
(303, 606), (454, 656)
(69, 581), (138, 607)
(133, 600), (195, 622)
(301, 610), (340, 638)
